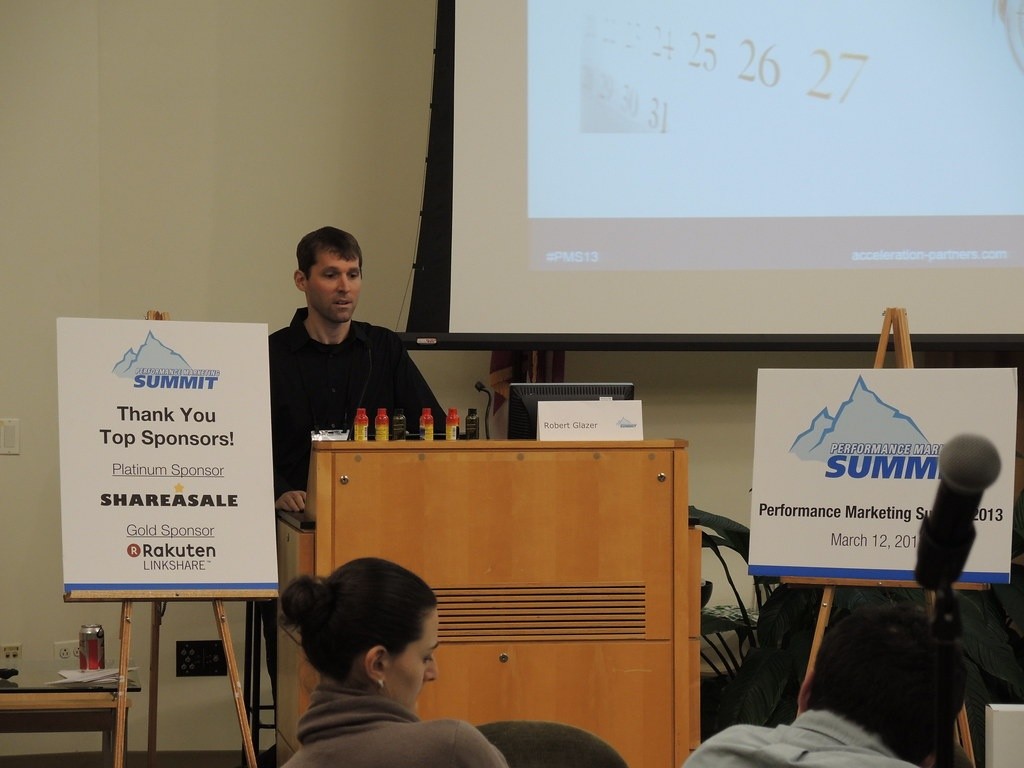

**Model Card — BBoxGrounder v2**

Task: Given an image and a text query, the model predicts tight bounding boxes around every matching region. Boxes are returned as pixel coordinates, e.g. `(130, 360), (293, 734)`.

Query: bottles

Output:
(375, 409), (389, 441)
(354, 408), (368, 441)
(466, 409), (479, 439)
(420, 408), (433, 440)
(446, 408), (460, 440)
(393, 408), (406, 441)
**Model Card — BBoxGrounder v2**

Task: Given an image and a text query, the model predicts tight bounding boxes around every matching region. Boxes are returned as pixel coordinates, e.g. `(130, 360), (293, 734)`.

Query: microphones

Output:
(347, 339), (374, 439)
(916, 431), (1002, 590)
(475, 382), (492, 439)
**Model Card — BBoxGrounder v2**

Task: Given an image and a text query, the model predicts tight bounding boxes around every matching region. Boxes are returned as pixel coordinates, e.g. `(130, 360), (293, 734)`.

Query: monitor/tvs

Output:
(507, 383), (635, 439)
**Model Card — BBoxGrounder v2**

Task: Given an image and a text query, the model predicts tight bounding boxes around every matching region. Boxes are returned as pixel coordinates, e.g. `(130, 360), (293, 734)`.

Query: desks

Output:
(0, 658), (142, 768)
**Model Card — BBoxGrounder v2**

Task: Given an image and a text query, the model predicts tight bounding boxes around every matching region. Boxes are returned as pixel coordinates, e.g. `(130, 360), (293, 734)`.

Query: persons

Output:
(674, 596), (980, 768)
(259, 225), (461, 739)
(274, 558), (511, 768)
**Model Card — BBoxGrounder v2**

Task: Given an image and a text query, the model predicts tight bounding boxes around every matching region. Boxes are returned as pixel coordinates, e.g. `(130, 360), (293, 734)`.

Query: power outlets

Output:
(1, 644), (22, 658)
(55, 640), (80, 660)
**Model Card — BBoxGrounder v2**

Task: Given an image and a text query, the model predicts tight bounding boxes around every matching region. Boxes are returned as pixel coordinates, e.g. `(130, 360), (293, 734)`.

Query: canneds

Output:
(79, 624), (105, 670)
